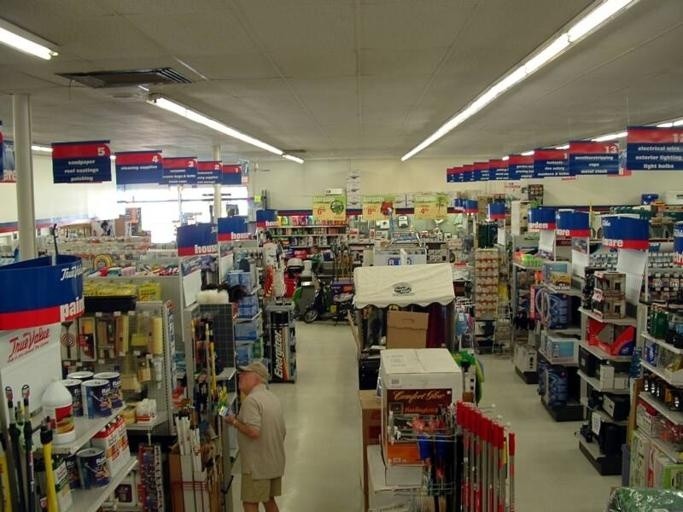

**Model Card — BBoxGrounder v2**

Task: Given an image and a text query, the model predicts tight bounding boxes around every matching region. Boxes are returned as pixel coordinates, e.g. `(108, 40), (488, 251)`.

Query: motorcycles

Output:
(303, 276), (354, 326)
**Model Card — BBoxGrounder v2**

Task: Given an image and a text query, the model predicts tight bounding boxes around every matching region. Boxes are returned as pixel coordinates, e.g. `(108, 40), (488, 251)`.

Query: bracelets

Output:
(232, 419), (237, 427)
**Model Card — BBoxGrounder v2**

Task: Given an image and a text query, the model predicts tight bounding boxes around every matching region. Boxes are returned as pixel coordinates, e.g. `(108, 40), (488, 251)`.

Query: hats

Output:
(237, 361), (271, 379)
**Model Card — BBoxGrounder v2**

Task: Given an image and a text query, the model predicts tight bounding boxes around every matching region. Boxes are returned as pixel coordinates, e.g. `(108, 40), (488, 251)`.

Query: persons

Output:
(219, 362), (288, 512)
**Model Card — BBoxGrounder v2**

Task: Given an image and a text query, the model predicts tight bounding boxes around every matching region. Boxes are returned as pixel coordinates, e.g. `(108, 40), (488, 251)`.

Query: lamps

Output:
(0, 18), (61, 60)
